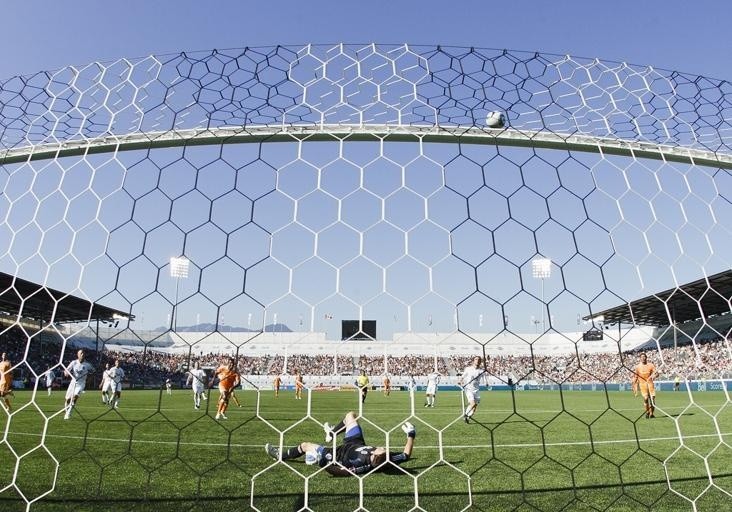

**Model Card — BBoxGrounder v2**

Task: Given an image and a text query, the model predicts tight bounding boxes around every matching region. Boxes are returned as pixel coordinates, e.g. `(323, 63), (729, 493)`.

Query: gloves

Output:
(402, 421), (415, 438)
(313, 449), (326, 467)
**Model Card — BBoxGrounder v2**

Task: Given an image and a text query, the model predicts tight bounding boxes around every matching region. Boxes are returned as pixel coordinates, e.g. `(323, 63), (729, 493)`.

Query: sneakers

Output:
(64, 412), (72, 419)
(324, 422), (334, 442)
(463, 416), (470, 423)
(216, 412), (227, 419)
(646, 412), (654, 418)
(265, 443), (280, 461)
(425, 404), (434, 408)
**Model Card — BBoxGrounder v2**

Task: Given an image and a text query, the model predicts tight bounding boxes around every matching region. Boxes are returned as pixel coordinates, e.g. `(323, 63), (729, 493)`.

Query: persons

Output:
(265, 412), (415, 478)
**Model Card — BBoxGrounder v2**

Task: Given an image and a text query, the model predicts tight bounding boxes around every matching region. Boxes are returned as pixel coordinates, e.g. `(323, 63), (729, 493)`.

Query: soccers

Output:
(486, 111), (506, 128)
